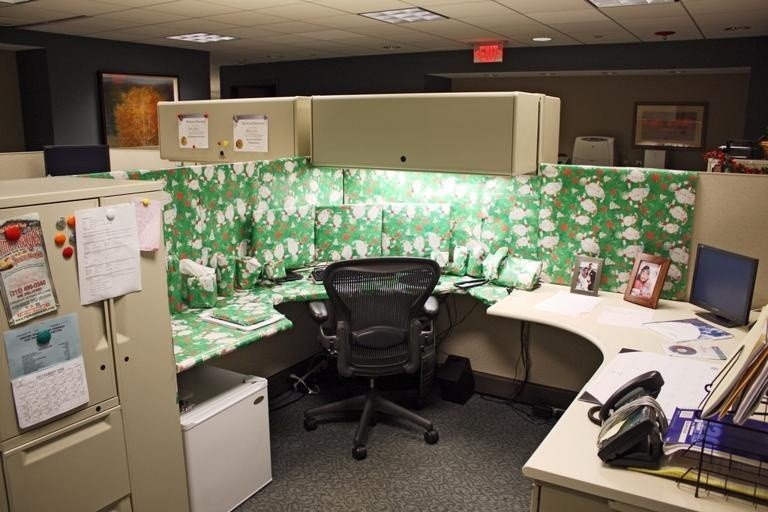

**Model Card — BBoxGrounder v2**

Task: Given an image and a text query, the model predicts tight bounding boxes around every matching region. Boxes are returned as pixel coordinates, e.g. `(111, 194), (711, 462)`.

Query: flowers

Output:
(702, 150), (758, 174)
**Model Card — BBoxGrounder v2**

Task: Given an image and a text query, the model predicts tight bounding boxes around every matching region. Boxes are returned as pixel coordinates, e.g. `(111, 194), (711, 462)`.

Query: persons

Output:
(633, 266), (650, 295)
(575, 263), (595, 291)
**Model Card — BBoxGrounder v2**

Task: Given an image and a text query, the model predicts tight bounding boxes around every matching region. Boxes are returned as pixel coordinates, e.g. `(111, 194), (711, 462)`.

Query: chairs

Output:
(304, 252), (442, 460)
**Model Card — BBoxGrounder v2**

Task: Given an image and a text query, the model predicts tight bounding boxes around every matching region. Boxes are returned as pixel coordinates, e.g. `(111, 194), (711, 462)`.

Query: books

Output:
(642, 304), (768, 486)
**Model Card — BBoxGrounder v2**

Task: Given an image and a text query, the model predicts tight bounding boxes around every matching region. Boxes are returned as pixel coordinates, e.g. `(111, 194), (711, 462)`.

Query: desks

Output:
(484, 282), (765, 512)
(165, 267), (515, 374)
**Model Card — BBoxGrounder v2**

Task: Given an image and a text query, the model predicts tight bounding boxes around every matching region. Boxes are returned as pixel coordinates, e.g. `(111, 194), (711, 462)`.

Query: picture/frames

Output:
(706, 157), (727, 172)
(630, 100), (710, 151)
(623, 251), (672, 310)
(570, 255), (604, 297)
(96, 69), (181, 149)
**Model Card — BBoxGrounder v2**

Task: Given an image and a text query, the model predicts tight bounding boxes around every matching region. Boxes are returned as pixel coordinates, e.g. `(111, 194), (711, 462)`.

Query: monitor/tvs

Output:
(689, 243), (759, 328)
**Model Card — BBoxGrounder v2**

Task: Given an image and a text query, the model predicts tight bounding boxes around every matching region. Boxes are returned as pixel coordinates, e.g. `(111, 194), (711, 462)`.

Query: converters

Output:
(534, 404), (552, 419)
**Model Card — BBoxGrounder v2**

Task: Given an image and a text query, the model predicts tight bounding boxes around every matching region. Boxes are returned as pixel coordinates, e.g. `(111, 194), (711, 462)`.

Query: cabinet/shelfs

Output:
(537, 92), (562, 163)
(311, 91), (540, 173)
(1, 180), (190, 512)
(154, 95), (312, 163)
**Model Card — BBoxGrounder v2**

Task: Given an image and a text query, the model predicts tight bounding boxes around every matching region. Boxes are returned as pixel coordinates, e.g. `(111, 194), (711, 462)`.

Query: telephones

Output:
(600, 371), (664, 469)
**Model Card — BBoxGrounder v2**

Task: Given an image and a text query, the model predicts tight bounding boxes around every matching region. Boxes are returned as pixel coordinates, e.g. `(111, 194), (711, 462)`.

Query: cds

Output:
(669, 346), (696, 355)
